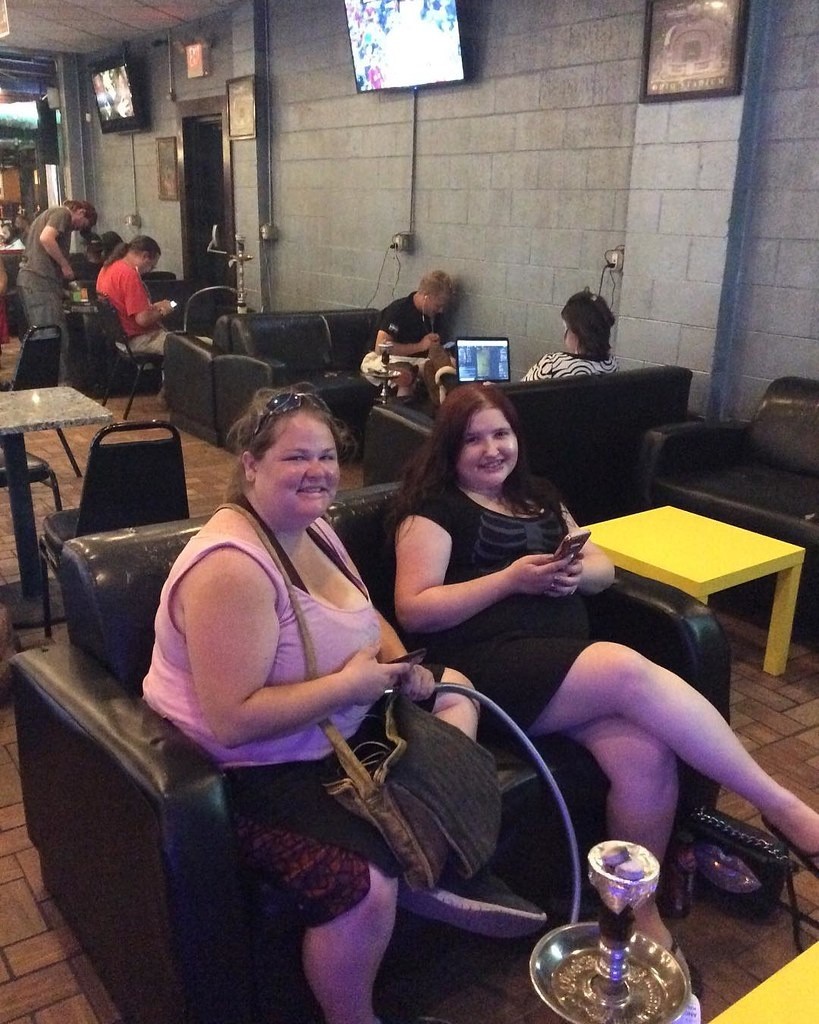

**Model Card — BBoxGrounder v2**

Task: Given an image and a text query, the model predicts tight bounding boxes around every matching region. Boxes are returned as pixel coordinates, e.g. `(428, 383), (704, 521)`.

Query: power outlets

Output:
(261, 225), (277, 239)
(393, 232), (413, 251)
(125, 215), (141, 226)
(605, 251), (623, 271)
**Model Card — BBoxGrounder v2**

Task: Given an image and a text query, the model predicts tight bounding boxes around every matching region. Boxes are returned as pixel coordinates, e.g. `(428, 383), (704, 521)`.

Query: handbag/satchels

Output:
(662, 801), (819, 953)
(321, 686), (502, 892)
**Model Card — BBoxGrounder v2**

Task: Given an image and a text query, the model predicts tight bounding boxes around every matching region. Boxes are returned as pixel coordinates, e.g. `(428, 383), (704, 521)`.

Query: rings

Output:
(568, 585), (577, 595)
(384, 685), (395, 694)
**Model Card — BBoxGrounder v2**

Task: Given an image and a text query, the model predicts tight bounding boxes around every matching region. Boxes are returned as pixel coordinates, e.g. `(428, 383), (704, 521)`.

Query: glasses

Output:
(148, 259), (156, 271)
(250, 392), (331, 453)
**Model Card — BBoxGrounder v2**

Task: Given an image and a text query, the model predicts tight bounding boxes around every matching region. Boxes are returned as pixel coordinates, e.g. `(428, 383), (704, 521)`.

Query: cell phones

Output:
(383, 648), (425, 664)
(170, 300), (180, 308)
(552, 530), (592, 562)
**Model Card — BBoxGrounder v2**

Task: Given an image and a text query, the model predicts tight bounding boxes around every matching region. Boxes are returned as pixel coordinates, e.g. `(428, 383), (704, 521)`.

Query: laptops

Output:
(456, 335), (511, 388)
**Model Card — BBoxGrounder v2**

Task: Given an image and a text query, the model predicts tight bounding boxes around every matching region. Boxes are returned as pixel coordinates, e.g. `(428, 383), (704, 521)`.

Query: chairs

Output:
(39, 421), (190, 638)
(0, 448), (62, 512)
(10, 325), (82, 478)
(90, 289), (163, 421)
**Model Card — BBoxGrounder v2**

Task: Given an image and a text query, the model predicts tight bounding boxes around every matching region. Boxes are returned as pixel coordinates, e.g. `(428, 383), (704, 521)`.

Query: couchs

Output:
(10, 483), (730, 1024)
(627, 377), (819, 638)
(60, 271), (694, 527)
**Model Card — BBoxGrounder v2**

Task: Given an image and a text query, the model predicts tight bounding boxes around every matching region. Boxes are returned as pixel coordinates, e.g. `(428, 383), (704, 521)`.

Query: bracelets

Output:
(153, 304), (165, 317)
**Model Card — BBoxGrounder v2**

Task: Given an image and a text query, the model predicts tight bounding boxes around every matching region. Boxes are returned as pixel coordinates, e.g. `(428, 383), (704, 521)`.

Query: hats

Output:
(63, 199), (98, 227)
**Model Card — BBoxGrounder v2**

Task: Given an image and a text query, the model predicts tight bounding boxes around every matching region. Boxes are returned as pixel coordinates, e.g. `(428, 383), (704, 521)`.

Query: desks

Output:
(581, 505), (806, 676)
(0, 387), (113, 629)
(709, 941), (819, 1024)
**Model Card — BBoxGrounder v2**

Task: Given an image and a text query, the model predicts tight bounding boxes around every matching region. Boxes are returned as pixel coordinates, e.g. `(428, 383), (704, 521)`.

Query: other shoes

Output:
(670, 937), (703, 996)
(760, 811), (819, 879)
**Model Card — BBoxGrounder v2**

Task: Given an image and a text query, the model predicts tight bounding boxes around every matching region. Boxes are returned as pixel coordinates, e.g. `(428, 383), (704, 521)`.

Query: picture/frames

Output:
(226, 74), (257, 140)
(154, 136), (178, 201)
(638, 0), (748, 104)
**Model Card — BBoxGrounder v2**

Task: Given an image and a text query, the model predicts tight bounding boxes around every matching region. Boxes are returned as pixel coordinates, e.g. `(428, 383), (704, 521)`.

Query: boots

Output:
(428, 343), (457, 385)
(424, 360), (450, 408)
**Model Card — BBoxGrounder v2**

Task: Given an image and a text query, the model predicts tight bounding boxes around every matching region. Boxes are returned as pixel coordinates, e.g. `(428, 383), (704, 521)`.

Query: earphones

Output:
(423, 295), (428, 301)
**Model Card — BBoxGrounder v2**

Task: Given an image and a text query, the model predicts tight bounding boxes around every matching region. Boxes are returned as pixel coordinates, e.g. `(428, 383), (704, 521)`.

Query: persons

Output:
(94, 68), (135, 120)
(96, 235), (188, 412)
(348, 0), (465, 91)
(16, 198), (98, 389)
(0, 215), (31, 354)
(520, 285), (619, 382)
(140, 380), (547, 1024)
(341, 270), (456, 446)
(381, 381), (819, 995)
(69, 230), (124, 285)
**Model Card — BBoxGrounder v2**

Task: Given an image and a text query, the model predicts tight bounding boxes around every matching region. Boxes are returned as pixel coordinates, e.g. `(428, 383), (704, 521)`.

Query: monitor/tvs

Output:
(88, 54), (144, 134)
(343, 0), (466, 94)
